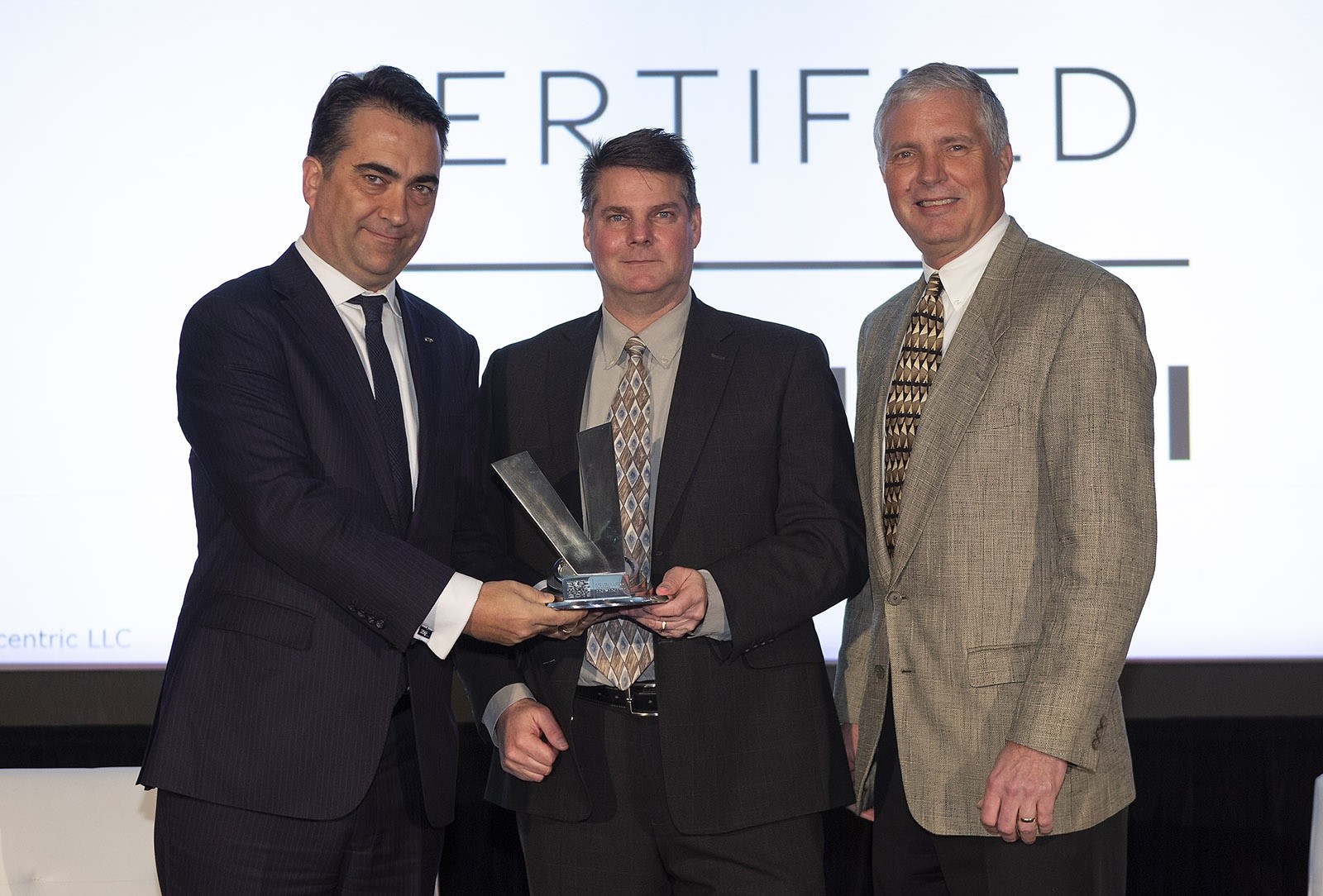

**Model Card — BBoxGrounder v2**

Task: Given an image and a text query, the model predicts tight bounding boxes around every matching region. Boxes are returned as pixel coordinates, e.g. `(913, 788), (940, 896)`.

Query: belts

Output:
(578, 681), (658, 716)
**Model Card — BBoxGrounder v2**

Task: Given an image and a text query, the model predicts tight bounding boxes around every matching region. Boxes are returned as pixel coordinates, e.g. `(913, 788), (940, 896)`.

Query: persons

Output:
(832, 63), (1156, 896)
(136, 66), (621, 896)
(453, 129), (870, 896)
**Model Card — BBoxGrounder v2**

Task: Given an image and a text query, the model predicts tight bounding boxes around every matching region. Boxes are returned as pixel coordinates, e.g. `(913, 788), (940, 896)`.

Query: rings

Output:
(559, 627), (574, 633)
(662, 621), (666, 633)
(1018, 816), (1036, 822)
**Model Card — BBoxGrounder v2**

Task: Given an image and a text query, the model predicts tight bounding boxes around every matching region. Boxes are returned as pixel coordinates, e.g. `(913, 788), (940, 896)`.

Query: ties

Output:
(347, 293), (413, 537)
(882, 274), (945, 564)
(586, 337), (654, 691)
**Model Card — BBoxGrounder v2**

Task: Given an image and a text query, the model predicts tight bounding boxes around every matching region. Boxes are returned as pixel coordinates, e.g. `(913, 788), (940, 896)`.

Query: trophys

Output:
(491, 422), (675, 613)
